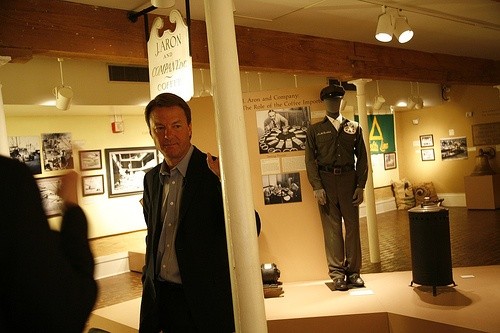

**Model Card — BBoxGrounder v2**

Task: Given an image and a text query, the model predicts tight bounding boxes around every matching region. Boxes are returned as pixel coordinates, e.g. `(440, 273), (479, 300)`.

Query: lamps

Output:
(407, 81), (423, 111)
(393, 8), (413, 43)
(374, 80), (385, 110)
(375, 5), (396, 42)
(52, 58), (73, 110)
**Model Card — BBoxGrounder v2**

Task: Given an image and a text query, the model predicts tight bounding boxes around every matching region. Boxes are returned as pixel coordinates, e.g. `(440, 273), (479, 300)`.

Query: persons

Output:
(264, 109), (289, 132)
(139, 92), (261, 333)
(0, 154), (100, 333)
(305, 84), (369, 291)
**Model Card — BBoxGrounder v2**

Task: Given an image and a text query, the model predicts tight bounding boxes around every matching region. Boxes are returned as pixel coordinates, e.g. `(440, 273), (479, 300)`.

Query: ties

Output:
(272, 120), (277, 127)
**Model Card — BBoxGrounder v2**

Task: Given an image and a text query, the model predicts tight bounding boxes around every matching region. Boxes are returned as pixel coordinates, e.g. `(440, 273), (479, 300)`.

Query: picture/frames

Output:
(384, 152), (397, 170)
(79, 150), (102, 171)
(421, 149), (435, 161)
(82, 175), (104, 197)
(35, 175), (79, 218)
(105, 146), (159, 198)
(420, 134), (434, 148)
